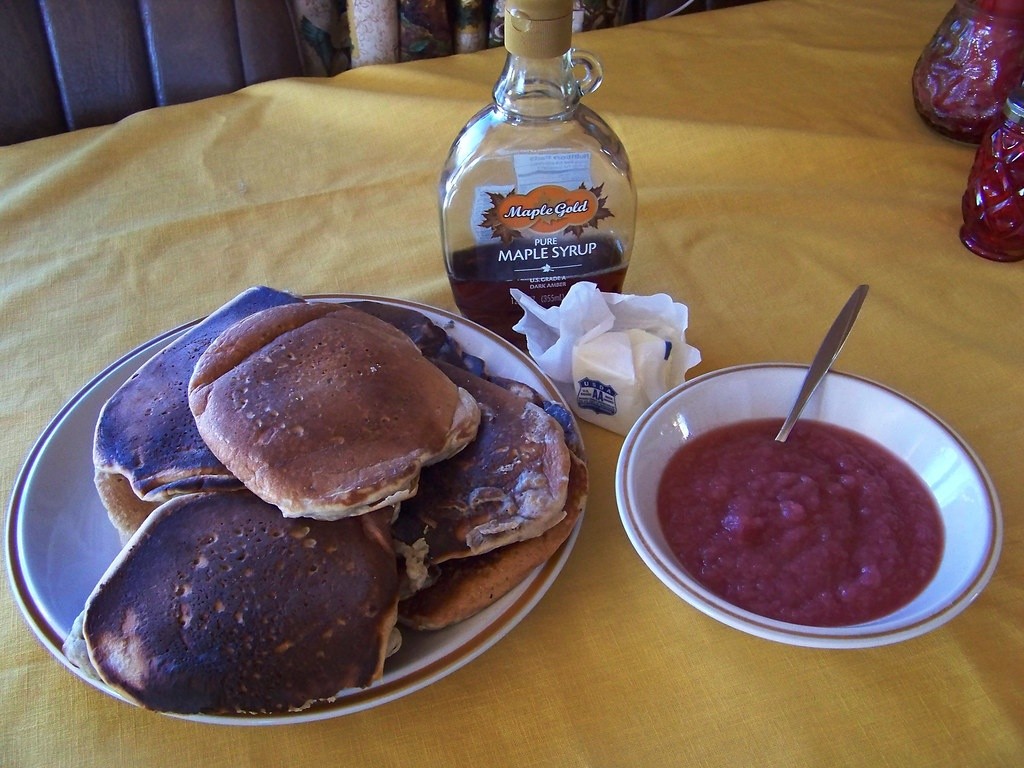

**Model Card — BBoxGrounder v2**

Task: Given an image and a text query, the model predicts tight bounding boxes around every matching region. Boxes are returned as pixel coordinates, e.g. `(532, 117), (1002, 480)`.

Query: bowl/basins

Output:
(616, 363), (1003, 648)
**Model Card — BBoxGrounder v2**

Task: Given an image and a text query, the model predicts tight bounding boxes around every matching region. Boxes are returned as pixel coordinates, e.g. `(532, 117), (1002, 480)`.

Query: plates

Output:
(5, 296), (587, 725)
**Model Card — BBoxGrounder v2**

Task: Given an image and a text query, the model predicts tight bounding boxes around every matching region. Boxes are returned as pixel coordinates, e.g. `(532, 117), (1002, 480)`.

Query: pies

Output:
(56, 284), (589, 714)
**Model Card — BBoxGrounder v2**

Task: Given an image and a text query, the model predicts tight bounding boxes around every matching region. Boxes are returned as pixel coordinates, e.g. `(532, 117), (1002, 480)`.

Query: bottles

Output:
(440, 1), (636, 349)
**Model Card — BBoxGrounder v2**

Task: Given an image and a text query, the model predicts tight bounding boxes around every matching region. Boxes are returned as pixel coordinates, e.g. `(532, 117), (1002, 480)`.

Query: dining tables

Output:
(0, 1), (1022, 768)
